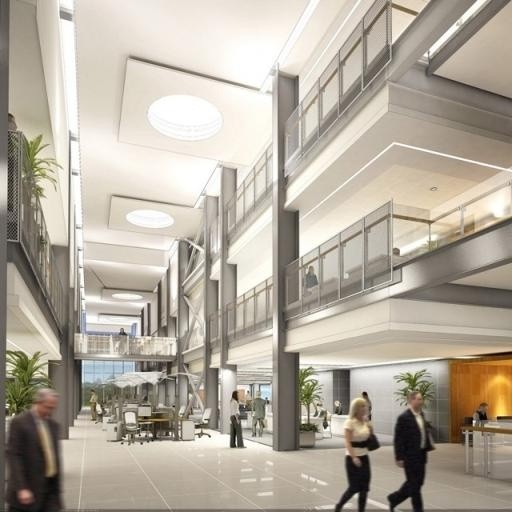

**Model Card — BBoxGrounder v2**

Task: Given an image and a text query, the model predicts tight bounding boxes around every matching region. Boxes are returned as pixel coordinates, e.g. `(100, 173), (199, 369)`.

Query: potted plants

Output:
(298, 366), (325, 448)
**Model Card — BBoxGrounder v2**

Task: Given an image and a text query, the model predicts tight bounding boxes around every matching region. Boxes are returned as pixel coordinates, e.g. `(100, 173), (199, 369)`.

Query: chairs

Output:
(103, 394), (213, 446)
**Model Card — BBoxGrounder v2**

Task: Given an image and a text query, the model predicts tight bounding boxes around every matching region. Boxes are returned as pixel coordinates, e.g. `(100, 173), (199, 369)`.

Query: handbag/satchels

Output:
(368, 435), (378, 451)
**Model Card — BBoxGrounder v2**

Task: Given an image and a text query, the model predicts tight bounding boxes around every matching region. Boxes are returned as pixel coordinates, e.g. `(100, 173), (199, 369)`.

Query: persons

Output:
(471, 402), (489, 420)
(229, 390), (246, 448)
(249, 390), (266, 438)
(335, 398), (373, 511)
(334, 400), (343, 415)
(89, 389), (98, 421)
(302, 265), (319, 290)
(265, 397), (270, 404)
(313, 402), (329, 429)
(119, 328), (127, 335)
(387, 391), (433, 511)
(361, 391), (372, 422)
(4, 388), (64, 511)
